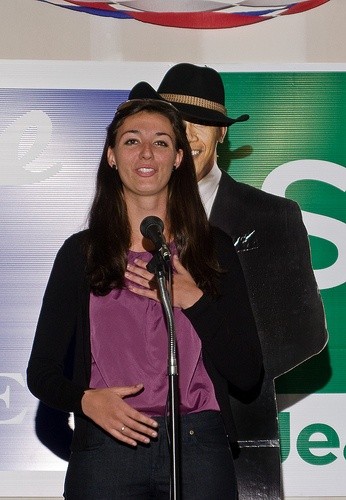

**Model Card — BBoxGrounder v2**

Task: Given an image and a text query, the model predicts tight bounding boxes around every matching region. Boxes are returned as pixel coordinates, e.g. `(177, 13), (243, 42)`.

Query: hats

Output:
(128, 63), (250, 125)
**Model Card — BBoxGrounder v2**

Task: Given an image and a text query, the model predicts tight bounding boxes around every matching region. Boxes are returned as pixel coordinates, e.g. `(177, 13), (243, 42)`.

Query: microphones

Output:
(140, 215), (171, 264)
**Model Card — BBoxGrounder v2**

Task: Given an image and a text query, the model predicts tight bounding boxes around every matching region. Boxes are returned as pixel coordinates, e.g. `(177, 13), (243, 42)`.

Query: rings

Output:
(121, 426), (127, 433)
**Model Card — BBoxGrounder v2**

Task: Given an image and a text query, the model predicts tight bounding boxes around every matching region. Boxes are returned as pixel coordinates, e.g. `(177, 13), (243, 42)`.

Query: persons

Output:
(26, 82), (266, 500)
(154, 63), (333, 500)
